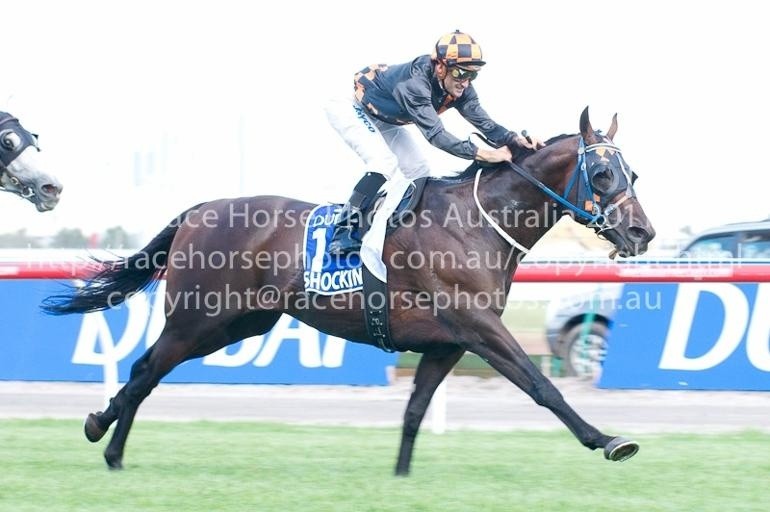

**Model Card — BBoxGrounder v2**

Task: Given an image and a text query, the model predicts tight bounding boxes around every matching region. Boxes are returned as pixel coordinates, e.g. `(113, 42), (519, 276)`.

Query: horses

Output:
(0, 110), (63, 212)
(37, 106), (656, 476)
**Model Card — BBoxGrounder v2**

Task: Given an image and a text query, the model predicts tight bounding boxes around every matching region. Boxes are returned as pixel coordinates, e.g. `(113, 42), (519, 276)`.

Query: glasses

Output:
(450, 63), (477, 82)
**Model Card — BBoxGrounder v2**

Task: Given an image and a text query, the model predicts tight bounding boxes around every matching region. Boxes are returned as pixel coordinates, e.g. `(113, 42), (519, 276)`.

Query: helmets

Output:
(432, 29), (486, 72)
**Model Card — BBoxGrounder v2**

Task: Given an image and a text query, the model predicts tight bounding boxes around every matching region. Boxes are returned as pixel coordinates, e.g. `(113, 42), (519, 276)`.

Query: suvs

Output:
(546, 217), (769, 381)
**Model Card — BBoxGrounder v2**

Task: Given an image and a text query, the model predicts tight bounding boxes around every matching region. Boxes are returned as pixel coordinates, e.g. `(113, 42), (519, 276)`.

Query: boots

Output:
(328, 189), (362, 255)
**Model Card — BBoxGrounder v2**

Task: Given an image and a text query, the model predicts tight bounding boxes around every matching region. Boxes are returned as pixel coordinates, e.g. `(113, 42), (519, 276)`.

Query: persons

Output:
(323, 29), (546, 257)
(743, 235), (769, 258)
